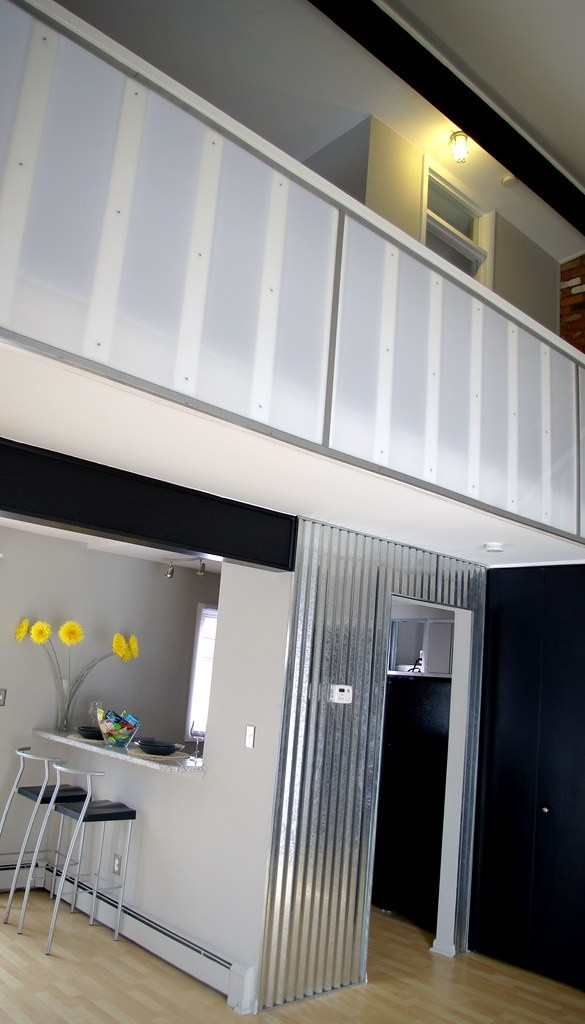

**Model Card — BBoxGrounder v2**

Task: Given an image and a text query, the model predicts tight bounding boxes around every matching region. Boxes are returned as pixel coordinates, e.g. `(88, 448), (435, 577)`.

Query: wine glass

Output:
(87, 700), (102, 725)
(190, 720), (206, 761)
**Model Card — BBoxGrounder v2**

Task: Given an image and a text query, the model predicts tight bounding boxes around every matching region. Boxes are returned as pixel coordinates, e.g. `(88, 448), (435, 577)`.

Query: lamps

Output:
(166, 560), (174, 579)
(450, 131), (468, 163)
(198, 559), (205, 576)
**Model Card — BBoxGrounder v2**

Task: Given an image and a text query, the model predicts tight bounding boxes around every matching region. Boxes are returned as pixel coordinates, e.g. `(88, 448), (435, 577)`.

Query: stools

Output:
(0, 747), (92, 924)
(17, 762), (136, 955)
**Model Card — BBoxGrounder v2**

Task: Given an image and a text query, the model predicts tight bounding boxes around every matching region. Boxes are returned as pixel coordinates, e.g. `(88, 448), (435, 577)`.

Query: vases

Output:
(56, 680), (84, 733)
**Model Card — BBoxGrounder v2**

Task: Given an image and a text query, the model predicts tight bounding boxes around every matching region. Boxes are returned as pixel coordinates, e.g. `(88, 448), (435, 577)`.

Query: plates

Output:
(135, 741), (185, 750)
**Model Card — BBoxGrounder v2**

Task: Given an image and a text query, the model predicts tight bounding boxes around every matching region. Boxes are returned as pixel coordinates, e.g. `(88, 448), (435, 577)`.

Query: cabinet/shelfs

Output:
(392, 620), (453, 674)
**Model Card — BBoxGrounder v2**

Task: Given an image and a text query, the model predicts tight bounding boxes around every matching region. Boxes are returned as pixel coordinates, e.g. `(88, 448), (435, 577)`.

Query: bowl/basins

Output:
(134, 740), (185, 755)
(77, 725), (104, 740)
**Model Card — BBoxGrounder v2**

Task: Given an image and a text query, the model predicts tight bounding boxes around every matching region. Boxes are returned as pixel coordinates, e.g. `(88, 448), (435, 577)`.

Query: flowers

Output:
(16, 619), (140, 728)
